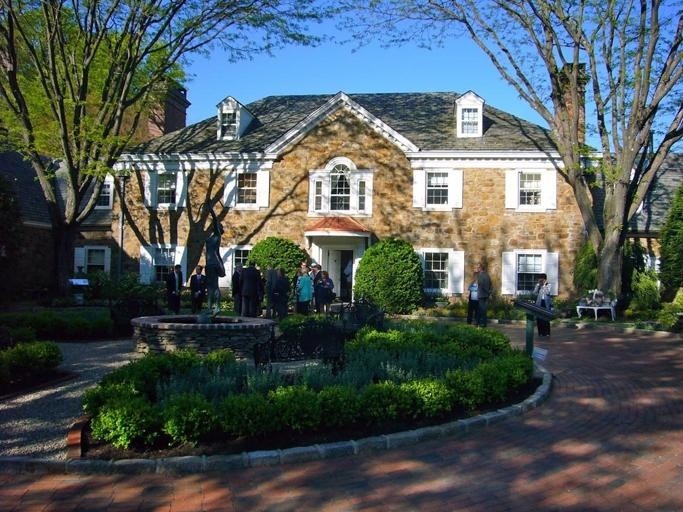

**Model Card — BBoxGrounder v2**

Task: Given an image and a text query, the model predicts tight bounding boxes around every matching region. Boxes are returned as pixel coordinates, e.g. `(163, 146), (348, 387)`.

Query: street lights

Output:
(113, 168), (129, 276)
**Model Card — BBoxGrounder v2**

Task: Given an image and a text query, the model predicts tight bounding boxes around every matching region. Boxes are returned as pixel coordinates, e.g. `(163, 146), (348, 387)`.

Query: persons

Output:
(230, 258), (352, 320)
(467, 272), (478, 324)
(167, 264), (182, 315)
(476, 262), (492, 326)
(190, 265), (207, 314)
(532, 274), (551, 337)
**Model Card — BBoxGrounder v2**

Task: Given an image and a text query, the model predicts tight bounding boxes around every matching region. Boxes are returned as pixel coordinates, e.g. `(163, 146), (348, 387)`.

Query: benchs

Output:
(574, 296), (618, 322)
